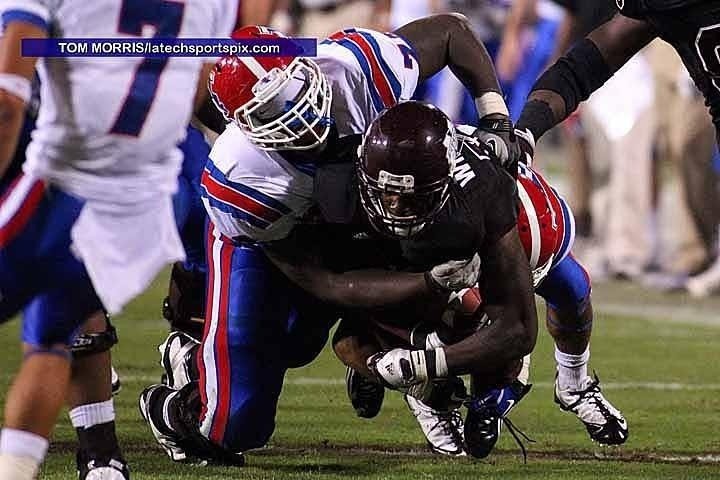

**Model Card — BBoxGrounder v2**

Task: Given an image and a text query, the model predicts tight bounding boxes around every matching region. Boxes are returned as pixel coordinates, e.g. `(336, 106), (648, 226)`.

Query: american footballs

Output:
(434, 286), (491, 344)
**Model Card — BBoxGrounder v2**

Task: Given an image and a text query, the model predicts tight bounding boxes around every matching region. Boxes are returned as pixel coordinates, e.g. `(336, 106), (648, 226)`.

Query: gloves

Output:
(366, 347), (466, 411)
(424, 252), (483, 292)
(477, 113), (538, 167)
(464, 378), (533, 419)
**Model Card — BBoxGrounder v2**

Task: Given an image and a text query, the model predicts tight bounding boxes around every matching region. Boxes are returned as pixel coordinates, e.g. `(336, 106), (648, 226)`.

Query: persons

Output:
(0, 0), (719, 480)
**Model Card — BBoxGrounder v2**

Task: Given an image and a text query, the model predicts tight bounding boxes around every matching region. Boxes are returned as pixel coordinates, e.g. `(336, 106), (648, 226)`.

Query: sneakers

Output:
(553, 365), (629, 448)
(111, 366), (122, 396)
(138, 331), (244, 466)
(344, 363), (384, 417)
(76, 451), (130, 480)
(402, 379), (509, 459)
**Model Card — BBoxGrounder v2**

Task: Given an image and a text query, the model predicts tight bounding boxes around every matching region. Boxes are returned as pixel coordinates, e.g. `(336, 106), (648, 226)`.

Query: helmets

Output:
(357, 101), (458, 193)
(206, 23), (306, 130)
